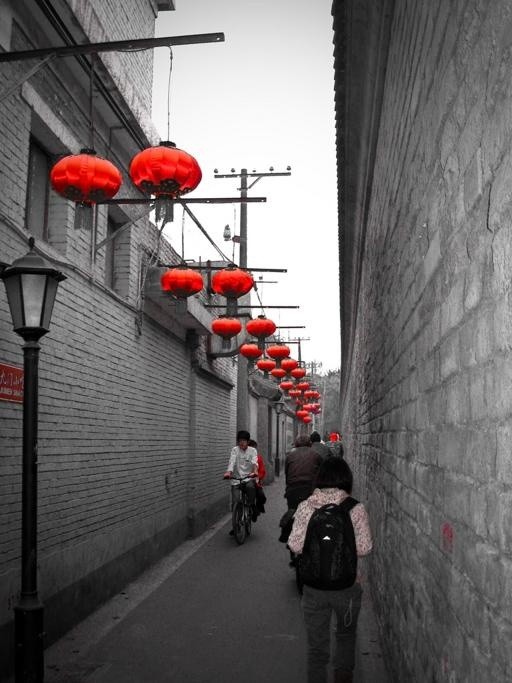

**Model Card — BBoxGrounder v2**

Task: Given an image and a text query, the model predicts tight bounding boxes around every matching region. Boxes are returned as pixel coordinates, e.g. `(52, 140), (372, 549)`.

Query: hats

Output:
(295, 435), (312, 447)
(330, 434), (337, 442)
(310, 433), (320, 442)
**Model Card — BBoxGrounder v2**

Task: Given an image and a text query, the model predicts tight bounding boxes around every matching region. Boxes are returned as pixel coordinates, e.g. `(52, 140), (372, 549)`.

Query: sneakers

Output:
(251, 513), (257, 522)
(229, 529), (235, 536)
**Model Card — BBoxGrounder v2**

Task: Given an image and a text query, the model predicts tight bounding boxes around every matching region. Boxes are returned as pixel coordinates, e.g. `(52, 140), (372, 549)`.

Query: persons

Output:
(247, 438), (267, 516)
(282, 429), (345, 568)
(222, 430), (260, 535)
(286, 455), (374, 683)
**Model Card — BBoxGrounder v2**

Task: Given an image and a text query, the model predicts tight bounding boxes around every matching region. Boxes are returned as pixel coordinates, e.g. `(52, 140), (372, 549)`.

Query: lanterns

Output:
(50, 147), (122, 230)
(160, 260), (204, 314)
(210, 262), (256, 316)
(211, 313), (322, 424)
(127, 139), (203, 223)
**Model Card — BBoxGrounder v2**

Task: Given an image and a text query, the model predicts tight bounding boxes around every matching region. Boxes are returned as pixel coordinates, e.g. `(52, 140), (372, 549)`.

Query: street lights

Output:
(0, 236), (68, 682)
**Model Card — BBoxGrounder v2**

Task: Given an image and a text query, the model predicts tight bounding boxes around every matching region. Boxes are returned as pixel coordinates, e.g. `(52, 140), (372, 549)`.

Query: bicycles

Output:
(222, 474), (257, 544)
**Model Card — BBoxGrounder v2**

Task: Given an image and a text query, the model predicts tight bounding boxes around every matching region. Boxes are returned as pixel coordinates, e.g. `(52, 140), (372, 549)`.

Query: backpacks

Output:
(299, 496), (360, 590)
(327, 441), (342, 458)
